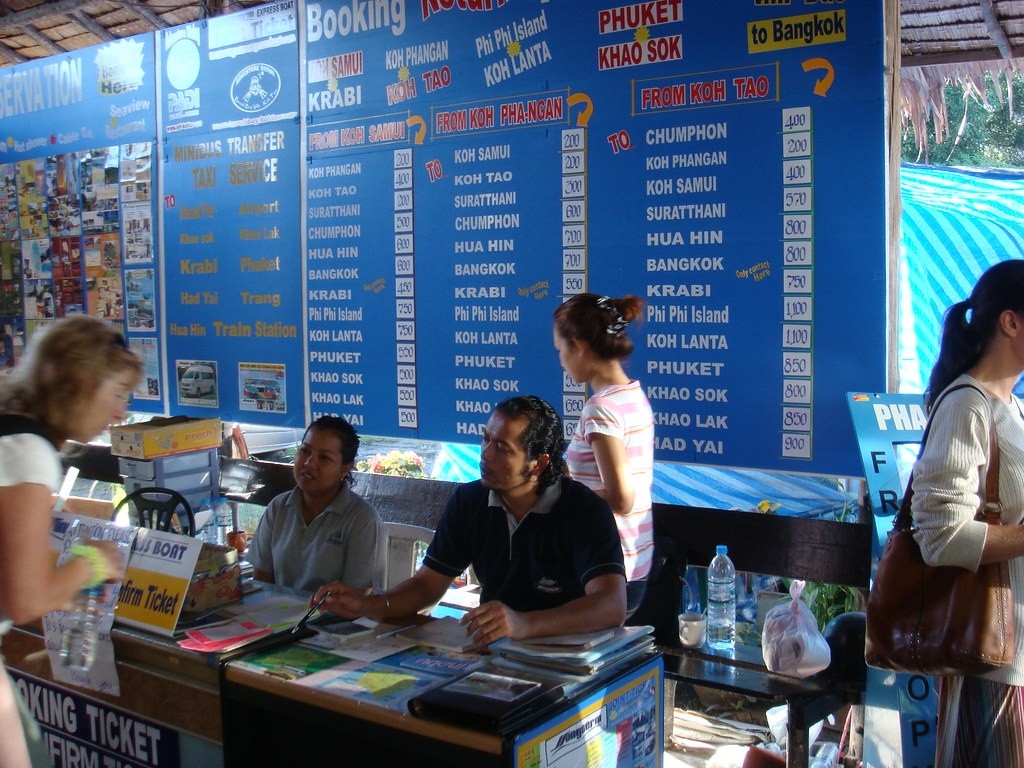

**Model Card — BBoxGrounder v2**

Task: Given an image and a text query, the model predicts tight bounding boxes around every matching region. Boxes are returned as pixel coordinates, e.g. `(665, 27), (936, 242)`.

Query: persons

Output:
(553, 294), (654, 620)
(0, 317), (143, 768)
(244, 417), (387, 599)
(911, 260), (1024, 768)
(308, 394), (627, 640)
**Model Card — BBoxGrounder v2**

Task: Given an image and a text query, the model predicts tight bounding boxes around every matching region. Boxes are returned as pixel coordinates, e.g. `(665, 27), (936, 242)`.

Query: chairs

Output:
(111, 487), (196, 538)
(381, 522), (435, 595)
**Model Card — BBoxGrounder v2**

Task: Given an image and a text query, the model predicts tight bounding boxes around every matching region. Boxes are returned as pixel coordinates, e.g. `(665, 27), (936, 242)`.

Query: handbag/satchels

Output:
(865, 384), (1015, 676)
(761, 580), (831, 679)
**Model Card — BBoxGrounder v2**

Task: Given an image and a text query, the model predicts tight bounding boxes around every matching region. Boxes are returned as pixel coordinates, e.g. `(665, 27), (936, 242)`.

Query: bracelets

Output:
(367, 594), (389, 620)
(68, 544), (108, 588)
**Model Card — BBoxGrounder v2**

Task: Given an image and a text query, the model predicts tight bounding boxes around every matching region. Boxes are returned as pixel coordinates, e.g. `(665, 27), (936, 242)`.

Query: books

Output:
(488, 627), (655, 683)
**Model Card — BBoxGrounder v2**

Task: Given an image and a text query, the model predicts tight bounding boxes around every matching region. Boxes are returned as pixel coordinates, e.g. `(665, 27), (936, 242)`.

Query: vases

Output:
(228, 531), (246, 552)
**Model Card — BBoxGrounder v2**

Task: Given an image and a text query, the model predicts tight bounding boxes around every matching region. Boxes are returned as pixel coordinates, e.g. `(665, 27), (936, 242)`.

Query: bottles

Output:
(59, 582), (103, 671)
(216, 497), (233, 546)
(811, 743), (837, 768)
(198, 498), (215, 544)
(707, 544), (736, 648)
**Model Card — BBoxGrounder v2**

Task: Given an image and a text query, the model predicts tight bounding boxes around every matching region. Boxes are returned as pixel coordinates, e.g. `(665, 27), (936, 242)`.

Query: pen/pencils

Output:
(290, 591), (332, 635)
(376, 624), (417, 639)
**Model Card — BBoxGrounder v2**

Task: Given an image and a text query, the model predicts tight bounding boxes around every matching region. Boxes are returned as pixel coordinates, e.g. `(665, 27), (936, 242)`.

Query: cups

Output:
(678, 613), (707, 648)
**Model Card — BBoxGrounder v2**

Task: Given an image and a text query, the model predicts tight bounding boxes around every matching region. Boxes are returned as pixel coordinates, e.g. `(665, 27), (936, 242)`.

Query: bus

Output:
(243, 378), (279, 401)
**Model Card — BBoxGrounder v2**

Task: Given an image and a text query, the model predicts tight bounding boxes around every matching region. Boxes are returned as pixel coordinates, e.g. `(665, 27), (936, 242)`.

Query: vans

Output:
(181, 366), (217, 398)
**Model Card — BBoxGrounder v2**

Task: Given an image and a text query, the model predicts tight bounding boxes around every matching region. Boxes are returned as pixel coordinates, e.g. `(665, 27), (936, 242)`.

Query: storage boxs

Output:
(110, 419), (223, 534)
(181, 543), (242, 612)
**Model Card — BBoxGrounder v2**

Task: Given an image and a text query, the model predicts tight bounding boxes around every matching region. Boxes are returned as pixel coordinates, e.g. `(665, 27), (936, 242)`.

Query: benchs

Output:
(57, 440), (871, 768)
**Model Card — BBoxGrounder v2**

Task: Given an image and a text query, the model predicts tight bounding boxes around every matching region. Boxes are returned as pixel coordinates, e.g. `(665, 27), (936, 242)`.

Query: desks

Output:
(0, 581), (664, 768)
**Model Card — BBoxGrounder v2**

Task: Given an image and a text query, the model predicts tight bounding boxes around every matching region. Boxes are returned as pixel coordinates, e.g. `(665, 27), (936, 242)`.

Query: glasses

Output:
(111, 333), (126, 349)
